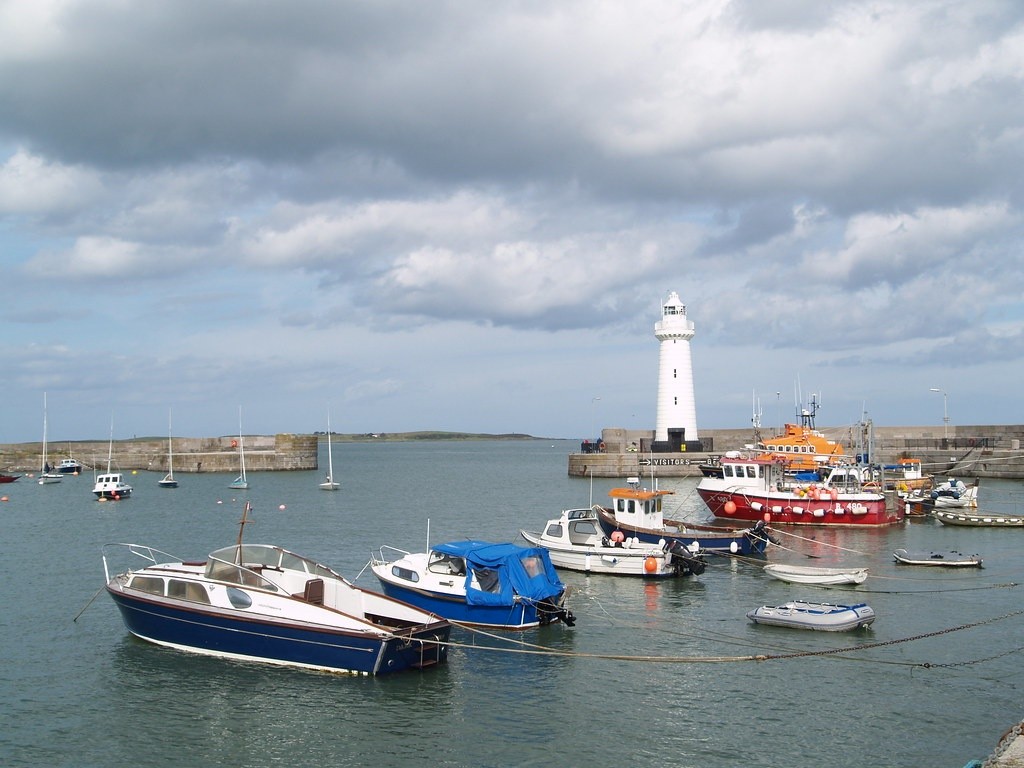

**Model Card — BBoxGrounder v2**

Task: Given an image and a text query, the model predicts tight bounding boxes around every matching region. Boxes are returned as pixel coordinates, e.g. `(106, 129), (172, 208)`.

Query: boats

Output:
(763, 564), (870, 585)
(520, 509), (708, 579)
(596, 478), (768, 556)
(745, 600), (875, 632)
(54, 459), (82, 474)
(884, 459), (935, 489)
(931, 508), (1024, 526)
(893, 549), (983, 566)
(905, 478), (980, 508)
(101, 501), (453, 677)
(370, 518), (577, 630)
(0, 472), (22, 483)
(696, 411), (937, 526)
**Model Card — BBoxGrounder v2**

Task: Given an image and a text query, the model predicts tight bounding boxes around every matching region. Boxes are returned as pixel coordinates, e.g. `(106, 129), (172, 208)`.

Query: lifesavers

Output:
(772, 455), (786, 460)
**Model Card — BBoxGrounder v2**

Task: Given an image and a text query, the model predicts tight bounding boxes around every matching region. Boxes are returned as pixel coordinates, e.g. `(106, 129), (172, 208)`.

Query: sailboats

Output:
(37, 392), (64, 484)
(158, 410), (178, 487)
(228, 409), (250, 488)
(319, 411), (340, 490)
(91, 417), (133, 498)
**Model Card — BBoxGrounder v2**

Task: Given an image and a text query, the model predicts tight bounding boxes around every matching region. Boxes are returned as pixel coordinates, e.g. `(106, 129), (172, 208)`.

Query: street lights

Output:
(592, 397), (601, 453)
(930, 388), (948, 438)
